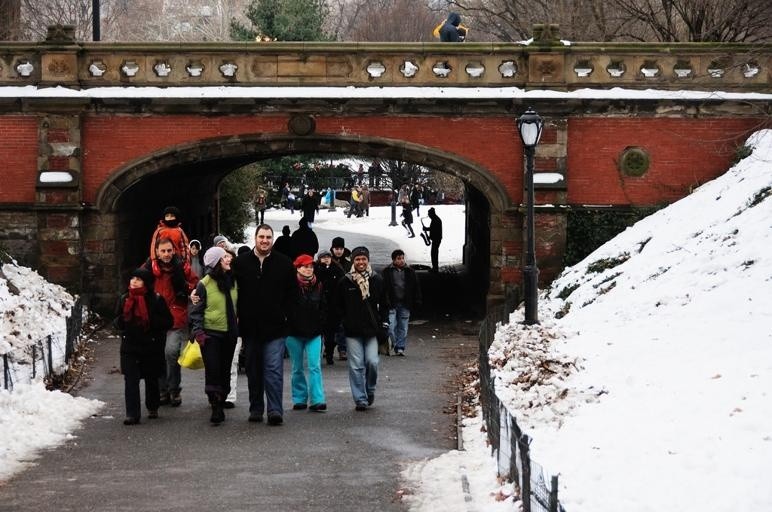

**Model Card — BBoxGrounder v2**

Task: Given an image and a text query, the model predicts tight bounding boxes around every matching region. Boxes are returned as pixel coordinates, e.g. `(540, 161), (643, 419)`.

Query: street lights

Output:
(515, 106), (546, 322)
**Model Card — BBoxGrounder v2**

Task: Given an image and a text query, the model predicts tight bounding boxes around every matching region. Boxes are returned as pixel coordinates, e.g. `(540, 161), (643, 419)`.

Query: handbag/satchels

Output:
(177, 337), (206, 370)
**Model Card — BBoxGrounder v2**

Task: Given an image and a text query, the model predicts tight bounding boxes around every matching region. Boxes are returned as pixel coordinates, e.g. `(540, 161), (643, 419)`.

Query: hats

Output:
(351, 246), (370, 263)
(318, 249), (332, 259)
(213, 235), (228, 246)
(293, 254), (314, 268)
(202, 246), (226, 268)
(163, 207), (180, 220)
(332, 237), (345, 248)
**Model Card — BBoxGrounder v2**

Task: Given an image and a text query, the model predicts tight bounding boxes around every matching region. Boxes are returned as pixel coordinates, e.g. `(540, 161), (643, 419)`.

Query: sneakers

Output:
(268, 413), (284, 424)
(356, 395), (374, 410)
(160, 391), (168, 405)
(248, 411), (263, 423)
(293, 403), (307, 410)
(169, 388), (182, 406)
(148, 410), (158, 420)
(309, 403), (326, 411)
(123, 417), (138, 425)
(208, 394), (234, 424)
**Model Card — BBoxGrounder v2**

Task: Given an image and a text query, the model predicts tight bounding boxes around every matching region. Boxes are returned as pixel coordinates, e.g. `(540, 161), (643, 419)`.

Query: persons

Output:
(433, 18), (470, 43)
(438, 11), (466, 42)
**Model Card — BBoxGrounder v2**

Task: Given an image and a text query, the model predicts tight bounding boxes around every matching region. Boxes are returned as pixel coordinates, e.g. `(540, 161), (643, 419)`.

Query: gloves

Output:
(194, 331), (209, 346)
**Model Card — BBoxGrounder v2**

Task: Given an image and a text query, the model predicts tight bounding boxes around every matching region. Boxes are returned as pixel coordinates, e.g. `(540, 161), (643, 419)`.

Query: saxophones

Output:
(420, 216), (431, 246)
